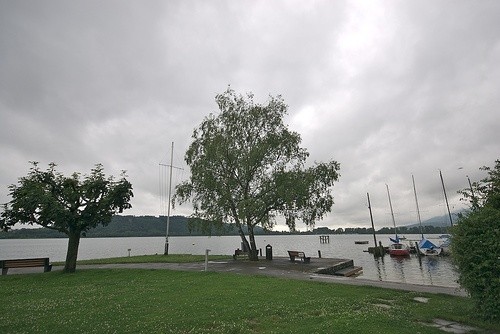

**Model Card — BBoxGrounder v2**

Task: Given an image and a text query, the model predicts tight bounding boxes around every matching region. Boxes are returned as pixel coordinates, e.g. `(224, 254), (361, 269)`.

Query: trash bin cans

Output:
(265, 244), (273, 261)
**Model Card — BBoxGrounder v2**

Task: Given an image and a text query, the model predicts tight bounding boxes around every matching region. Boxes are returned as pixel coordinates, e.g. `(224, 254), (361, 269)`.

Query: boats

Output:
(389, 242), (408, 256)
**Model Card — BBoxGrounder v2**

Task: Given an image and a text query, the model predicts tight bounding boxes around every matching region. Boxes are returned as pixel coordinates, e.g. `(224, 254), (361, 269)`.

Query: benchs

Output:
(287, 251), (307, 261)
(233, 249), (259, 258)
(0, 258), (53, 275)
